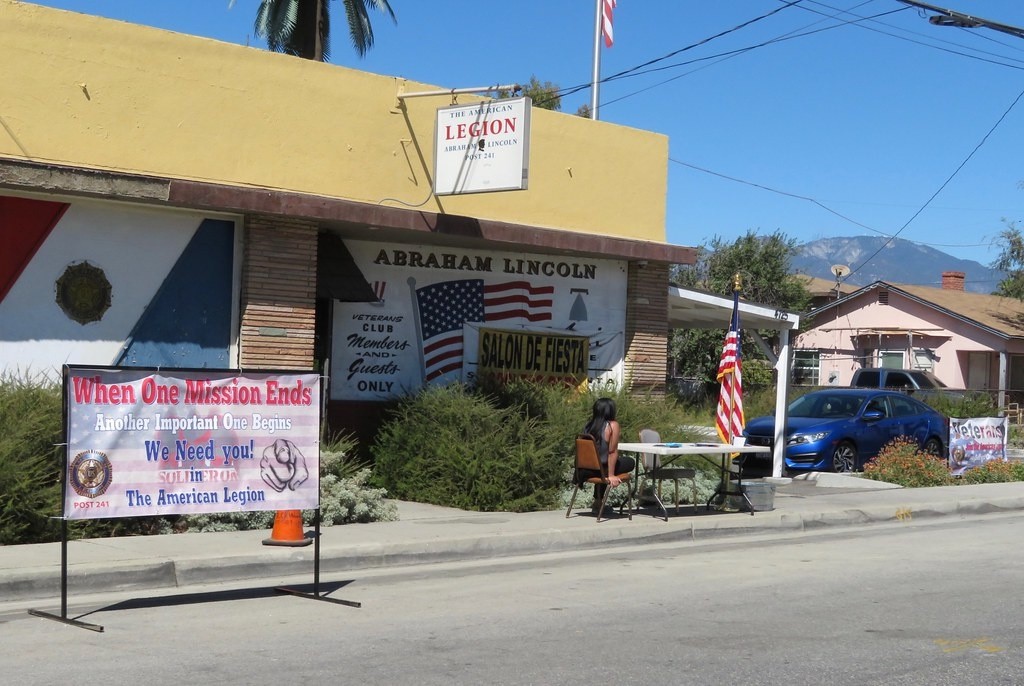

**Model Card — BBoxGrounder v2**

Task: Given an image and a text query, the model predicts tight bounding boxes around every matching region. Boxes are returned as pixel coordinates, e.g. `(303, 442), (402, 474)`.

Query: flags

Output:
(601, 0), (616, 48)
(716, 291), (745, 459)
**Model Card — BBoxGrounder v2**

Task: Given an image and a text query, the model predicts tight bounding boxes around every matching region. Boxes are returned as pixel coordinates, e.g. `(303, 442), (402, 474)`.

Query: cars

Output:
(742, 389), (949, 473)
(850, 368), (993, 413)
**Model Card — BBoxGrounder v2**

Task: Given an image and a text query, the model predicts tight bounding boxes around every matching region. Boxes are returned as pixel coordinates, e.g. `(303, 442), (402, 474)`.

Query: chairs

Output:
(638, 429), (698, 515)
(1007, 402), (1023, 424)
(566, 434), (634, 520)
(827, 400), (886, 418)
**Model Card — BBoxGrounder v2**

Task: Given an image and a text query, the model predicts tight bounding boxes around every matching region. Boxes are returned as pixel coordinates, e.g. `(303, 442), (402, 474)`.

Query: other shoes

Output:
(592, 504), (613, 514)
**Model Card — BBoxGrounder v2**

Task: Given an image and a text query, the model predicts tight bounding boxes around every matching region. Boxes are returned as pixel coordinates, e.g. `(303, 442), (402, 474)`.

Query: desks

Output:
(616, 443), (771, 522)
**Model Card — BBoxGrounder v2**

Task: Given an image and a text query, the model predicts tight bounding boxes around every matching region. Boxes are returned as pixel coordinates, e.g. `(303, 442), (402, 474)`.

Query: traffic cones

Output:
(261, 510), (313, 548)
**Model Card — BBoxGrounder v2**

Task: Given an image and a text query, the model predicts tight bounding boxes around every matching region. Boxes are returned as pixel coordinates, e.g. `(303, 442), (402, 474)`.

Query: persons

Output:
(572, 397), (635, 514)
